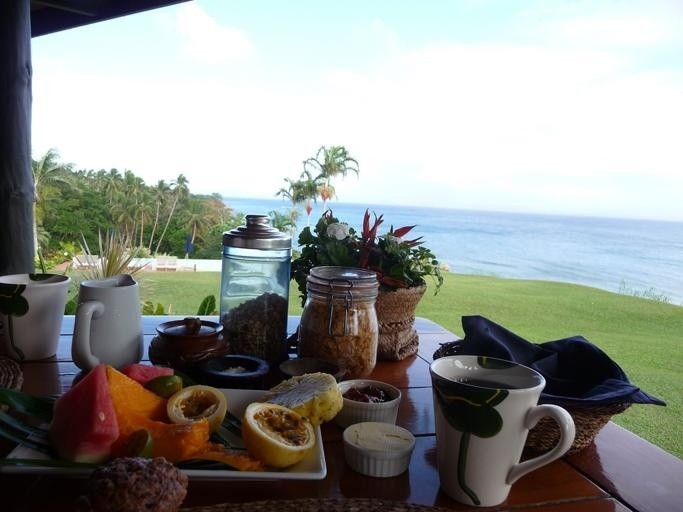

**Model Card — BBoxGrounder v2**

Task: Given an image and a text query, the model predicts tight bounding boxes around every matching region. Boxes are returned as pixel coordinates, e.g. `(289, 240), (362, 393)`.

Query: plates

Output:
(1, 386), (327, 481)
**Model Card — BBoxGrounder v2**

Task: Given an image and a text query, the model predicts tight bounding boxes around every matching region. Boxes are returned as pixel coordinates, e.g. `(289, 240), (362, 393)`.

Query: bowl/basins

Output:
(201, 353), (272, 386)
(335, 378), (402, 426)
(341, 420), (417, 479)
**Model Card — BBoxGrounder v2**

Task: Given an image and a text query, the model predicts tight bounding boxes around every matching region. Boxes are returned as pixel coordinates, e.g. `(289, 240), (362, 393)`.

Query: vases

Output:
(371, 285), (429, 363)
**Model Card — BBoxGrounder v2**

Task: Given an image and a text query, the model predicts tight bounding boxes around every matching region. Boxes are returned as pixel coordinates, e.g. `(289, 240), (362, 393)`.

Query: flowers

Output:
(288, 205), (453, 299)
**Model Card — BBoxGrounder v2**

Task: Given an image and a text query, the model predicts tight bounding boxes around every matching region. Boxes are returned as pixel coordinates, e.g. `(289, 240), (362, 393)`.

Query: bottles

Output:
(296, 264), (382, 376)
(218, 213), (290, 361)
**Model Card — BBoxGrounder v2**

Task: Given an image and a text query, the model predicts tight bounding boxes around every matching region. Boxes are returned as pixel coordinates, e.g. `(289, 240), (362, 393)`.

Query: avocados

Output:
(113, 429), (152, 460)
(146, 376), (183, 400)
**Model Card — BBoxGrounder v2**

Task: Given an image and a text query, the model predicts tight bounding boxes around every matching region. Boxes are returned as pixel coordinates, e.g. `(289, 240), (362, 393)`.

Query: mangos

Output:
(107, 365), (261, 472)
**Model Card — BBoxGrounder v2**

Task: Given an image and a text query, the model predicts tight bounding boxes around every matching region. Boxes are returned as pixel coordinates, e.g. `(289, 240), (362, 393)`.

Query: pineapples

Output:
(260, 372), (342, 427)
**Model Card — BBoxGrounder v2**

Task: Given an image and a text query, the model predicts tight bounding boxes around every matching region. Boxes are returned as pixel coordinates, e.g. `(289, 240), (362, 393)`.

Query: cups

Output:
(147, 316), (231, 375)
(426, 353), (574, 507)
(70, 271), (144, 369)
(0, 272), (68, 362)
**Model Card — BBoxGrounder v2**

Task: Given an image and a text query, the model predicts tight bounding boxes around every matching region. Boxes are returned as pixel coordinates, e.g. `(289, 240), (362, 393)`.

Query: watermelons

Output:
(125, 363), (172, 382)
(123, 366), (175, 386)
(52, 364), (120, 463)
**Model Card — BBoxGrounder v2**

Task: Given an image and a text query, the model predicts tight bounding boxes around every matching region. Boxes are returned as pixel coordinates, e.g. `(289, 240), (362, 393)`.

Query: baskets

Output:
(432, 339), (634, 457)
(294, 269), (427, 361)
